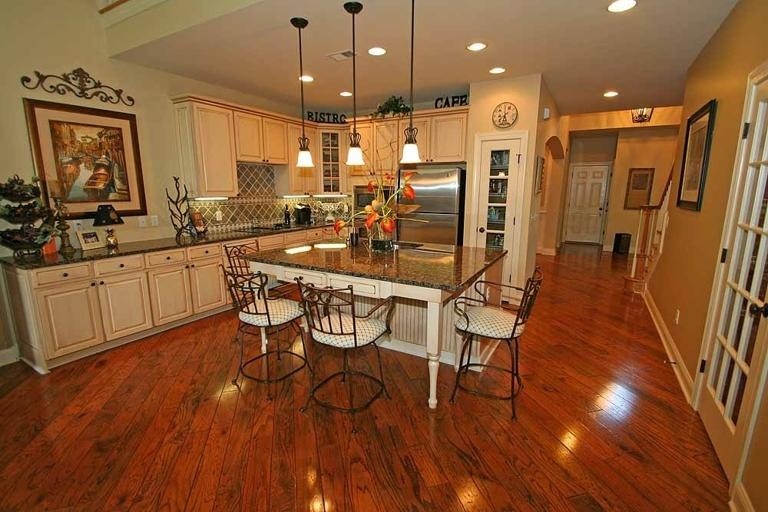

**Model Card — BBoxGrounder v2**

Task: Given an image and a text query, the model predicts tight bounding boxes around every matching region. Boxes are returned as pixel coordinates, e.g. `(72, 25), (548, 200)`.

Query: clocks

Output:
(490, 102), (520, 129)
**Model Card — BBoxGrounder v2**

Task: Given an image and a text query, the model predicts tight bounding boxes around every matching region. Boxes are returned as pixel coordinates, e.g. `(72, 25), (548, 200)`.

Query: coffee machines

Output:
(295, 207), (311, 224)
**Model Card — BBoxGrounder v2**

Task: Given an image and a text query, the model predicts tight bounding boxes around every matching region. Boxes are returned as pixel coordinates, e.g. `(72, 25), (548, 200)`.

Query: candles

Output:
(48, 179), (61, 196)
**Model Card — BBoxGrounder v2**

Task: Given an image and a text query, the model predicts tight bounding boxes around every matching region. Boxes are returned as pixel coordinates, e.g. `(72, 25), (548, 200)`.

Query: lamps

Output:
(288, 17), (317, 169)
(339, 0), (367, 167)
(399, 0), (424, 165)
(91, 204), (126, 253)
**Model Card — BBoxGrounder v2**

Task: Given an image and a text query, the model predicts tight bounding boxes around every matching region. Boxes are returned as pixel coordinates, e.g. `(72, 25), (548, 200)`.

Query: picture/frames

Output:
(631, 107), (655, 123)
(675, 98), (719, 213)
(75, 229), (106, 251)
(623, 167), (654, 211)
(535, 154), (545, 196)
(19, 97), (150, 220)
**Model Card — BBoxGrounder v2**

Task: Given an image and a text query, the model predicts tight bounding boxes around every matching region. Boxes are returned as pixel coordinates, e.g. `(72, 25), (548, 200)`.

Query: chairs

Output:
(216, 264), (316, 404)
(448, 264), (546, 420)
(222, 237), (294, 338)
(292, 275), (397, 419)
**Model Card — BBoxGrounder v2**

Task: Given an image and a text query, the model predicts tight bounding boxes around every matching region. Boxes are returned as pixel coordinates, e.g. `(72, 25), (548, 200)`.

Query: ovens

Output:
(354, 188), (390, 239)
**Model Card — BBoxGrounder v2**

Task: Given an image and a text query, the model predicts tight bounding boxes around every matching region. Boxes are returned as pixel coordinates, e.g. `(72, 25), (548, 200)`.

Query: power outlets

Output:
(73, 219), (84, 234)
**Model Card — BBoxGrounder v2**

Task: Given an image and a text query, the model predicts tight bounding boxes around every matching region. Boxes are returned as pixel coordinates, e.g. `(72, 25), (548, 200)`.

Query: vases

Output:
(370, 236), (395, 253)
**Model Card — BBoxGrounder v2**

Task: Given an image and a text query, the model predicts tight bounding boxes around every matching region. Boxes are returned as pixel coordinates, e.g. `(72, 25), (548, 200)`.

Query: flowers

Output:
(331, 169), (429, 241)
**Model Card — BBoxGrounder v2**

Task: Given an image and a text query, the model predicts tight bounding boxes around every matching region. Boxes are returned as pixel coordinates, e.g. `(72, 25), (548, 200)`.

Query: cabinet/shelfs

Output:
(398, 105), (469, 166)
(1, 251), (153, 376)
(221, 231), (286, 311)
(473, 134), (521, 308)
(143, 242), (227, 333)
(346, 112), (398, 178)
(170, 93), (239, 201)
(258, 225), (335, 248)
(317, 122), (349, 199)
(272, 116), (318, 196)
(232, 102), (289, 164)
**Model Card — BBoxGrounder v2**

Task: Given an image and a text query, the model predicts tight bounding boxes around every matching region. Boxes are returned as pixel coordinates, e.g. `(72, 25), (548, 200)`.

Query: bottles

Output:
(284, 204), (290, 224)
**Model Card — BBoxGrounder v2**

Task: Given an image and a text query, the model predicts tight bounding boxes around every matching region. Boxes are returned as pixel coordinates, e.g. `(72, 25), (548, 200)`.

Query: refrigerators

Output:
(395, 166), (463, 246)
